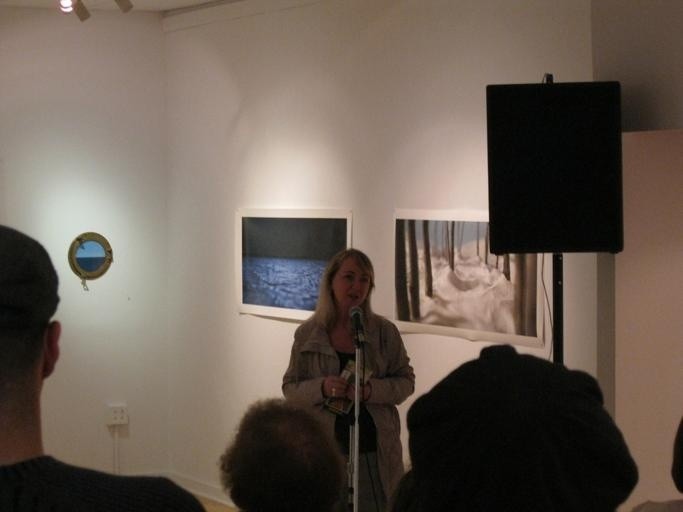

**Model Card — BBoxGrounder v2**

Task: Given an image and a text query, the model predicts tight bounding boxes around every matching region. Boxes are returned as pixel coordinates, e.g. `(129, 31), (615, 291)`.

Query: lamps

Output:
(59, 0), (74, 14)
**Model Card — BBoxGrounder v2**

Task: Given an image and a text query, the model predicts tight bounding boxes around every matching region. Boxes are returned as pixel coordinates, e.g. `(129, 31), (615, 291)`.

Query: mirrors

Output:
(69, 231), (112, 279)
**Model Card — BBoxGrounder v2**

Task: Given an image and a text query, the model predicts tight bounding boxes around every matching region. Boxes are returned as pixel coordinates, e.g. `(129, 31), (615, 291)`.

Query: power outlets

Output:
(106, 402), (129, 426)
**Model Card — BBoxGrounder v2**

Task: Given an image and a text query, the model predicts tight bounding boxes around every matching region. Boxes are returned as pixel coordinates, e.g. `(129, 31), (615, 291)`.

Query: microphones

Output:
(349, 306), (366, 345)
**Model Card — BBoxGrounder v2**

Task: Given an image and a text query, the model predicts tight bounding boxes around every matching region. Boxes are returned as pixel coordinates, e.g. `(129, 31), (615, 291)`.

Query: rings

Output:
(331, 393), (335, 397)
(331, 388), (335, 393)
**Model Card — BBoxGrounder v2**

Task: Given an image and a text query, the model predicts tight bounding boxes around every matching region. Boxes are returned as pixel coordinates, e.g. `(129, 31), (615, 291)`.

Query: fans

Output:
(72, 0), (134, 23)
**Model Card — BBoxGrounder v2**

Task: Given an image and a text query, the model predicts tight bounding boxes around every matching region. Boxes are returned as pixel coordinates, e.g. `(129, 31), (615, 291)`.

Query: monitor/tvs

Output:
(485, 79), (624, 256)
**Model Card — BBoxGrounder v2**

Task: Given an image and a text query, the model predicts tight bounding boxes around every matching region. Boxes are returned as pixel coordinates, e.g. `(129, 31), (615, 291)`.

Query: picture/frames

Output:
(234, 207), (354, 323)
(391, 207), (545, 351)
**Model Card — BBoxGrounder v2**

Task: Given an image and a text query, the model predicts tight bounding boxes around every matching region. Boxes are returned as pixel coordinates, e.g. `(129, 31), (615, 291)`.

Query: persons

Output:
(280, 247), (417, 512)
(1, 221), (208, 512)
(215, 394), (346, 512)
(633, 414), (682, 512)
